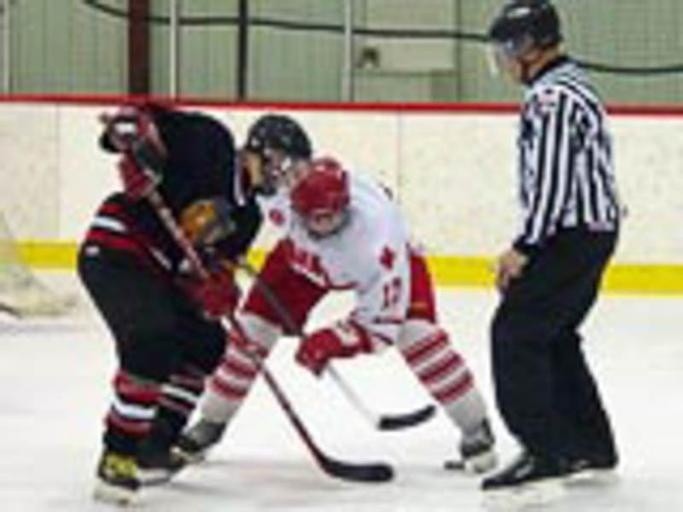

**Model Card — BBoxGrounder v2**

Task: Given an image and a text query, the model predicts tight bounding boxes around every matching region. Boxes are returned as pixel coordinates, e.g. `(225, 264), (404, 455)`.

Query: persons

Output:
(172, 153), (495, 458)
(477, 1), (622, 491)
(72, 99), (312, 491)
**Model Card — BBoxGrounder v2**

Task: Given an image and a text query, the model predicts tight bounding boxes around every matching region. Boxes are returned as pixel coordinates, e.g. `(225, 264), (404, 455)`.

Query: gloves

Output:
(189, 266), (241, 322)
(119, 113), (171, 202)
(294, 321), (371, 377)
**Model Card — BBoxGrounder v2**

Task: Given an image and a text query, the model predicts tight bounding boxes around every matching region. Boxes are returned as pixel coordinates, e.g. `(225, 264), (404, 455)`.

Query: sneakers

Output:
(182, 418), (224, 453)
(98, 440), (188, 487)
(461, 417), (493, 459)
(482, 443), (618, 492)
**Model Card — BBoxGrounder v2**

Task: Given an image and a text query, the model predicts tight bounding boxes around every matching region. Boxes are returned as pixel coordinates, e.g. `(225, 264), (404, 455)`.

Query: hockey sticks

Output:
(242, 261), (436, 430)
(97, 111), (393, 481)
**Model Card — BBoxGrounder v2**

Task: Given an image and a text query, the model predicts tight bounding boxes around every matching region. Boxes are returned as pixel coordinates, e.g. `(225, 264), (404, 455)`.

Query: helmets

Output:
(486, 0), (565, 51)
(288, 156), (352, 238)
(246, 113), (313, 196)
